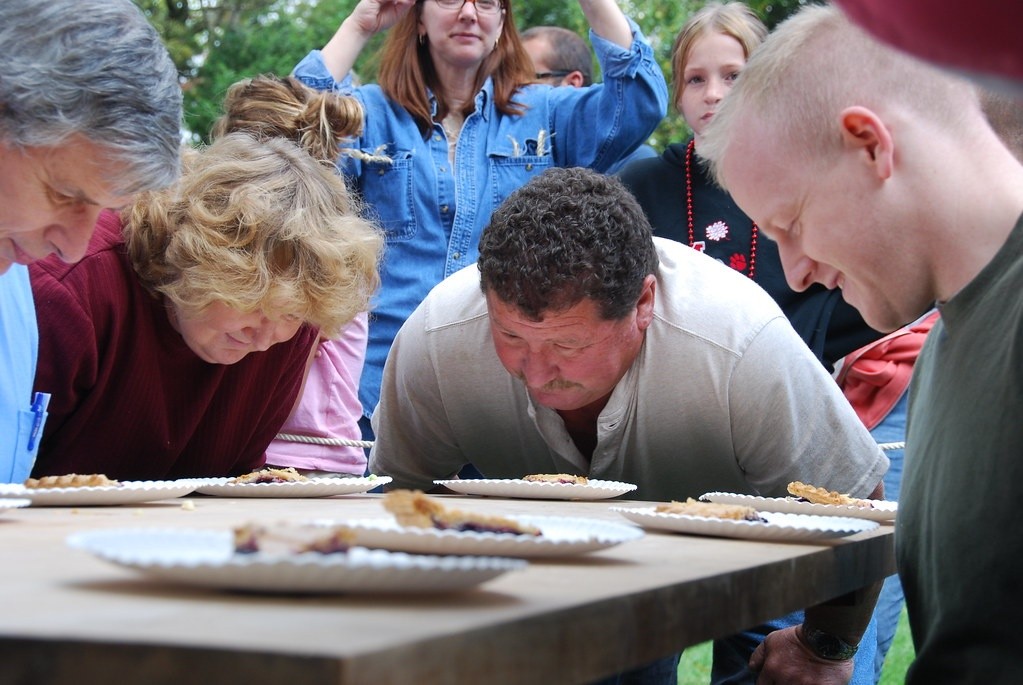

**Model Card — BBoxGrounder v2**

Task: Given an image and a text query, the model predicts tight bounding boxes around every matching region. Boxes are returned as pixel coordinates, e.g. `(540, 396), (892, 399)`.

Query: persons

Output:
(518, 0), (1023, 685)
(27, 131), (386, 483)
(212, 73), (369, 479)
(0, 0), (184, 484)
(368, 167), (890, 685)
(287, 0), (670, 495)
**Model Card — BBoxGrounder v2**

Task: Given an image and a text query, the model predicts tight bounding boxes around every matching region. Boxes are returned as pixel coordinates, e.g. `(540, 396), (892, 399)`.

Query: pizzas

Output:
(23, 467), (874, 555)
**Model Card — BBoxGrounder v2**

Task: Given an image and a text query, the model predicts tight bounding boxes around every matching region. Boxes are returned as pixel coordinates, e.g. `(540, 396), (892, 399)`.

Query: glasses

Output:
(436, 0), (504, 14)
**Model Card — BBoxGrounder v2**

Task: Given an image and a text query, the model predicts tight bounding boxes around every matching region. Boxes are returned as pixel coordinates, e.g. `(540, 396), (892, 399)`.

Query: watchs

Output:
(802, 620), (859, 660)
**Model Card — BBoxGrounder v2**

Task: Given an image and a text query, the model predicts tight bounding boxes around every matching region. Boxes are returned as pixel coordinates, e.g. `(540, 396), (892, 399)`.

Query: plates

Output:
(0, 497), (31, 516)
(0, 479), (210, 504)
(276, 514), (645, 557)
(432, 478), (638, 499)
(66, 526), (530, 590)
(193, 474), (394, 497)
(609, 505), (880, 540)
(698, 495), (898, 522)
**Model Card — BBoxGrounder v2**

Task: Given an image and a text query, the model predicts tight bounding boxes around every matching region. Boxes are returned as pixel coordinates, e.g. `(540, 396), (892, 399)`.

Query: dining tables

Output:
(0, 496), (897, 685)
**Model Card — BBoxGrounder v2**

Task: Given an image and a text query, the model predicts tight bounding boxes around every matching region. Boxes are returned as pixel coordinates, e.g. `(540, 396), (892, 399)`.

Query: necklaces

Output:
(685, 138), (759, 279)
